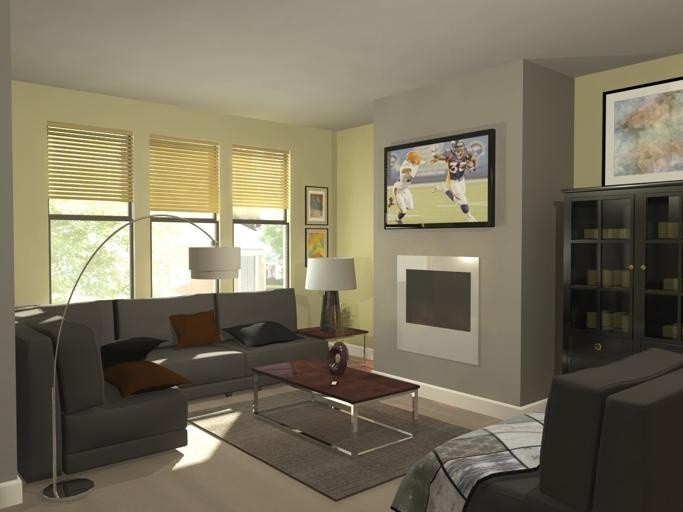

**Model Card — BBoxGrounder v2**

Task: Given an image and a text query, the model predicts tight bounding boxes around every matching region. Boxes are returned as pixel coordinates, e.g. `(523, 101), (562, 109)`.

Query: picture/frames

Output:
(601, 77), (683, 186)
(305, 229), (329, 269)
(305, 186), (327, 225)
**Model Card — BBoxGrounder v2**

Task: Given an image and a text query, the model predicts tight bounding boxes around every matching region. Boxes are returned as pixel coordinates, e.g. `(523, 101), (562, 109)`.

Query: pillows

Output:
(105, 361), (192, 398)
(171, 310), (221, 351)
(221, 321), (303, 348)
(101, 335), (169, 370)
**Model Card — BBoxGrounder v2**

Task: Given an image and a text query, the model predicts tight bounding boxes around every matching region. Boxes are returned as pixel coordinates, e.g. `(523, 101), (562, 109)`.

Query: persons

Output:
(431, 139), (478, 222)
(387, 151), (426, 224)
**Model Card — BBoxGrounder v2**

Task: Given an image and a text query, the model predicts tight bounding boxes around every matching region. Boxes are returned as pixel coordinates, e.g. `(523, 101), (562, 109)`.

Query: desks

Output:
(252, 359), (420, 457)
(303, 326), (369, 371)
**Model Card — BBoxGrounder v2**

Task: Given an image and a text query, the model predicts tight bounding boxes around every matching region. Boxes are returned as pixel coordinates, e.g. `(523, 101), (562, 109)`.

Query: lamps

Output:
(42, 214), (240, 500)
(305, 257), (359, 332)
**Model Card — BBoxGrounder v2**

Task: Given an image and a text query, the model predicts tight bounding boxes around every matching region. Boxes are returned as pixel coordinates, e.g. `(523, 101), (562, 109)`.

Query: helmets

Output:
(450, 139), (464, 158)
(406, 151), (420, 165)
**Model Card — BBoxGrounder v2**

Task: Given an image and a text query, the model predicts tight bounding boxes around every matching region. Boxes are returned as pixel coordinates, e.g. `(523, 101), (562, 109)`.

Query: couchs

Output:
(391, 347), (682, 510)
(15, 288), (329, 483)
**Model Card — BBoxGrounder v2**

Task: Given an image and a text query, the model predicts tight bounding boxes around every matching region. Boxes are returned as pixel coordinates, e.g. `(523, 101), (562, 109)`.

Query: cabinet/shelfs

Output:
(560, 180), (682, 374)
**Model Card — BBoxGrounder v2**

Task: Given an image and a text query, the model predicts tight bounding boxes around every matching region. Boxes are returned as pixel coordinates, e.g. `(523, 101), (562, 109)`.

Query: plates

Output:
(584, 229), (631, 239)
(587, 269), (630, 288)
(586, 310), (630, 333)
(658, 222), (678, 239)
(663, 279), (678, 290)
(662, 324), (678, 339)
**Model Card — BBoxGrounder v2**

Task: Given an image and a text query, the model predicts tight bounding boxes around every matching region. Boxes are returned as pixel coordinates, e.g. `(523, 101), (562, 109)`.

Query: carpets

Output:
(188, 390), (473, 501)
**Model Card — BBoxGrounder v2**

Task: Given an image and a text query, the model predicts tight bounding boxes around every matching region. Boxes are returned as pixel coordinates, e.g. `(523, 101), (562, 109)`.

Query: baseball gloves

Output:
(464, 157), (478, 172)
